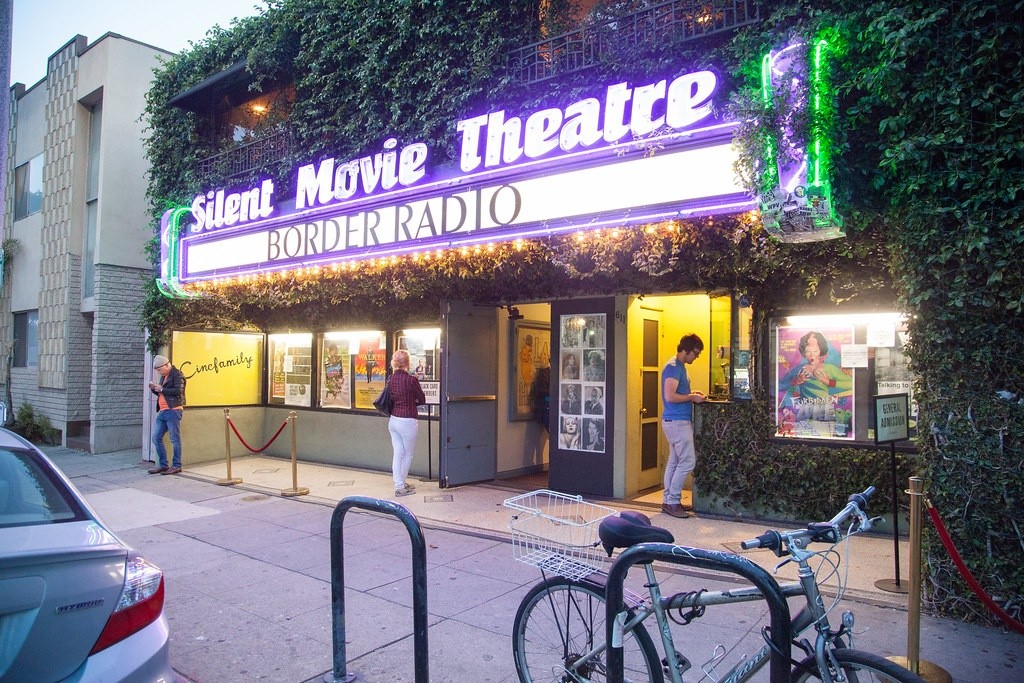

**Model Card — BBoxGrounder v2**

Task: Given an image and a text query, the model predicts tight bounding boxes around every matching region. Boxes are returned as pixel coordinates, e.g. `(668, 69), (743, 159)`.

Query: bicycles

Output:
(503, 486), (926, 683)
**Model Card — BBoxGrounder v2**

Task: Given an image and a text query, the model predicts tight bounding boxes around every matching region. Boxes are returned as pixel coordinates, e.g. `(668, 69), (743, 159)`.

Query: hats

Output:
(153, 355), (170, 369)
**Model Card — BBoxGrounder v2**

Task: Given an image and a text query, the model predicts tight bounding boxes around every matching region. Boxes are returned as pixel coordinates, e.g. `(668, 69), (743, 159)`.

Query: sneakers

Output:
(662, 503), (692, 518)
(148, 466), (169, 474)
(160, 466), (182, 474)
(395, 483), (416, 497)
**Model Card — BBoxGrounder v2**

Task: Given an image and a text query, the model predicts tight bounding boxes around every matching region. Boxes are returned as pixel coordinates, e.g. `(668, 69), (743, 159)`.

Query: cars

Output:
(0, 427), (174, 683)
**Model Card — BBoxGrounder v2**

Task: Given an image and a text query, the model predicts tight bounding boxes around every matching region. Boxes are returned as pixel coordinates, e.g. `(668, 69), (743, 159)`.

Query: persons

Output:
(325, 344), (343, 400)
(660, 332), (708, 519)
(560, 316), (605, 451)
(533, 356), (549, 432)
(148, 354), (186, 475)
(387, 350), (426, 496)
(366, 345), (375, 383)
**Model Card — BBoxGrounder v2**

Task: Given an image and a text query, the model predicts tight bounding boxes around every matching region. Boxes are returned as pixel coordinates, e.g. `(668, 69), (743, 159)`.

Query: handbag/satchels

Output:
(373, 375), (394, 417)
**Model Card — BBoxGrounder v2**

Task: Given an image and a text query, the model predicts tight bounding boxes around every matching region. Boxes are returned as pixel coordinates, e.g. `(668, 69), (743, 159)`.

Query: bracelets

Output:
(161, 387), (163, 391)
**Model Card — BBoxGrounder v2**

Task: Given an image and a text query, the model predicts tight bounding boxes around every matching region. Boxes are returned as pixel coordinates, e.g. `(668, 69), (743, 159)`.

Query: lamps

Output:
(739, 293), (752, 308)
(363, 120), (399, 151)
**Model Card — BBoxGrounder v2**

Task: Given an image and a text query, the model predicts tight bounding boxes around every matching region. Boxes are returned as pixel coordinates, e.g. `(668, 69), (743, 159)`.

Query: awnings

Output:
(167, 53), (293, 117)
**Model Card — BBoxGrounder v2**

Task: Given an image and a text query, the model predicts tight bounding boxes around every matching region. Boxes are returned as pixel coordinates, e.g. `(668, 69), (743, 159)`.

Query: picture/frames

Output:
(508, 316), (551, 421)
(398, 335), (435, 381)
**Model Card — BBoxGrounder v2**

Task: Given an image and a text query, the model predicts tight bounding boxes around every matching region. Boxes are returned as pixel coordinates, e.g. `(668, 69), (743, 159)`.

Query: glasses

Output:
(692, 350), (699, 356)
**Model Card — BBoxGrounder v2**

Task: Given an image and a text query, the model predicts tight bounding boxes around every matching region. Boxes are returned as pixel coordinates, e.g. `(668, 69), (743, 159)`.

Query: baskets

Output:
(503, 489), (617, 582)
